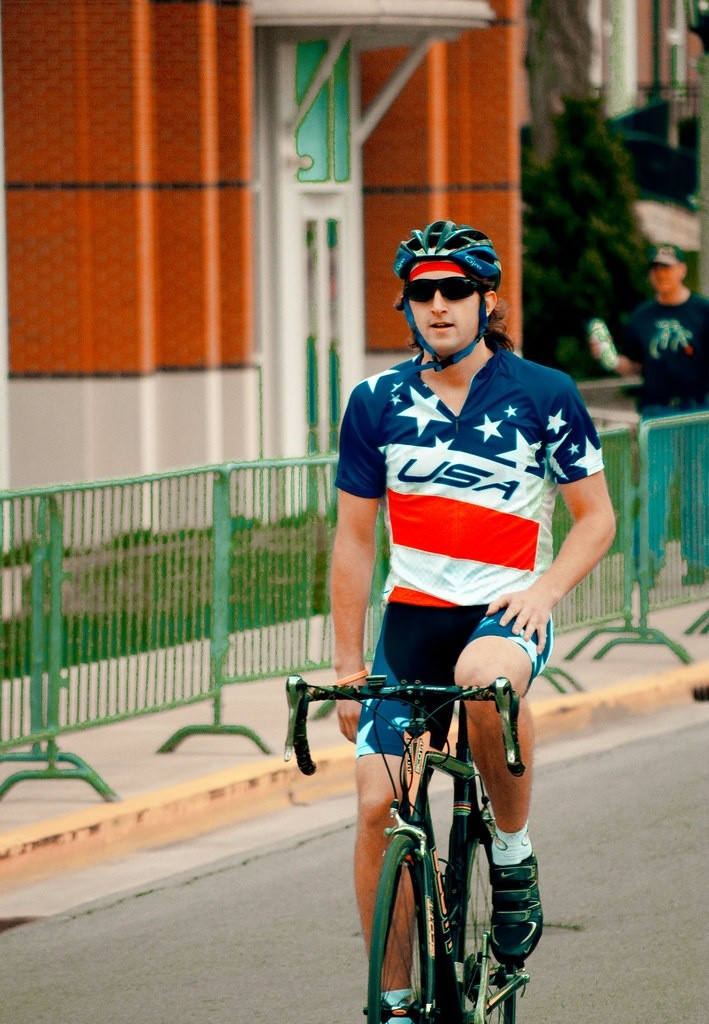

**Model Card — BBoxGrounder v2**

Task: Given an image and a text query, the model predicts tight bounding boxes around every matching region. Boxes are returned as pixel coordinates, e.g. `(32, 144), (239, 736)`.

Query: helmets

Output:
(392, 220), (502, 291)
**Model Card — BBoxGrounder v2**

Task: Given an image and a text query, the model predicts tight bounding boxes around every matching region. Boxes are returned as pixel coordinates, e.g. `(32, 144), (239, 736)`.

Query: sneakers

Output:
(489, 848), (543, 964)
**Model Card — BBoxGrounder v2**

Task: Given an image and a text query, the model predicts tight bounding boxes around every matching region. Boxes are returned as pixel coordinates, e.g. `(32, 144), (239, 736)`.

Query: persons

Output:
(591, 245), (709, 423)
(331, 222), (615, 1024)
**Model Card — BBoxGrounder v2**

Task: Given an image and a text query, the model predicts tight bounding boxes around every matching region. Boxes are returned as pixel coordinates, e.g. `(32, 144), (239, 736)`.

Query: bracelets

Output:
(339, 671), (368, 685)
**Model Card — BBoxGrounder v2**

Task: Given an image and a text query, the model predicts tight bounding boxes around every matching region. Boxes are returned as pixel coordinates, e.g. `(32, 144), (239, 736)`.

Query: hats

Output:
(648, 243), (687, 266)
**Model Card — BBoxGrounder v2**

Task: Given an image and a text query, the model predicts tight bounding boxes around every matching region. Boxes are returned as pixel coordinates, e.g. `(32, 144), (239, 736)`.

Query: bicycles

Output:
(260, 665), (541, 1024)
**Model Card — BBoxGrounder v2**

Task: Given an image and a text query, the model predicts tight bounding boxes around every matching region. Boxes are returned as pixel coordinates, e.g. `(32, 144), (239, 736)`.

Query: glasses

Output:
(404, 276), (489, 302)
